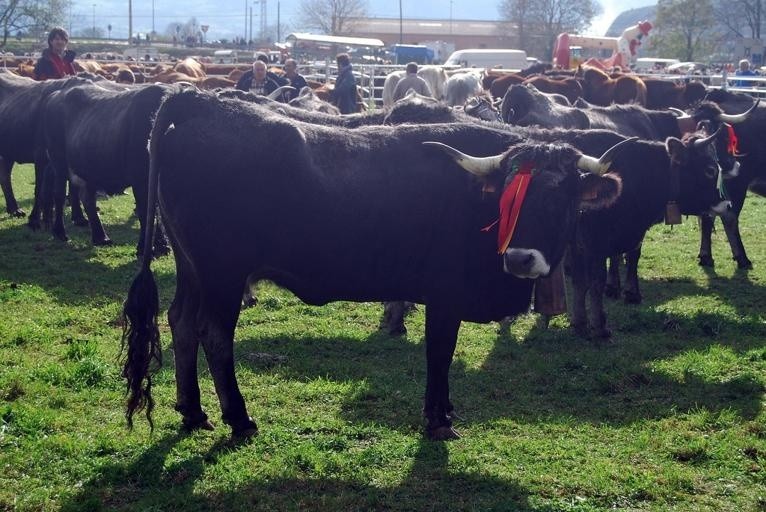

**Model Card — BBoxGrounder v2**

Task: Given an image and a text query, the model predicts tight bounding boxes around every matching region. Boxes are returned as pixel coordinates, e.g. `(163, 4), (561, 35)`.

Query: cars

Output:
(669, 62), (709, 73)
(526, 57), (541, 67)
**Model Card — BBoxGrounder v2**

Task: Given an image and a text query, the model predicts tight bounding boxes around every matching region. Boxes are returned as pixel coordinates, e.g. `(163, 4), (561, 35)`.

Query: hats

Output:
(638, 21), (651, 36)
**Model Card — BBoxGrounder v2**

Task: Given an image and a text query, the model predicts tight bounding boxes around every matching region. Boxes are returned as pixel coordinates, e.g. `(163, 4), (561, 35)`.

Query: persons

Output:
(144, 33), (151, 46)
(180, 36), (186, 48)
(232, 58), (238, 64)
(733, 59), (757, 88)
(84, 54), (91, 59)
(280, 59), (308, 94)
(136, 34), (141, 45)
(30, 28), (76, 78)
(358, 61), (387, 103)
(240, 59), (286, 104)
(602, 58), (725, 88)
(236, 55), (283, 91)
(392, 61), (435, 102)
(142, 54), (154, 62)
(95, 51), (137, 62)
(330, 52), (357, 114)
(116, 69), (136, 86)
(217, 59), (224, 65)
(172, 36), (177, 49)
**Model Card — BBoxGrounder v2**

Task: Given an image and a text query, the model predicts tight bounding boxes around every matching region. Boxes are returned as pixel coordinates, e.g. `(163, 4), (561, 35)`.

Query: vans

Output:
(445, 49), (527, 69)
(637, 57), (680, 71)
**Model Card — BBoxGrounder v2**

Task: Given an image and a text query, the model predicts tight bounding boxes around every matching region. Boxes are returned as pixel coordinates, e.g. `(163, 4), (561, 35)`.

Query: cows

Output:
(116, 85), (642, 448)
(0, 58), (766, 342)
